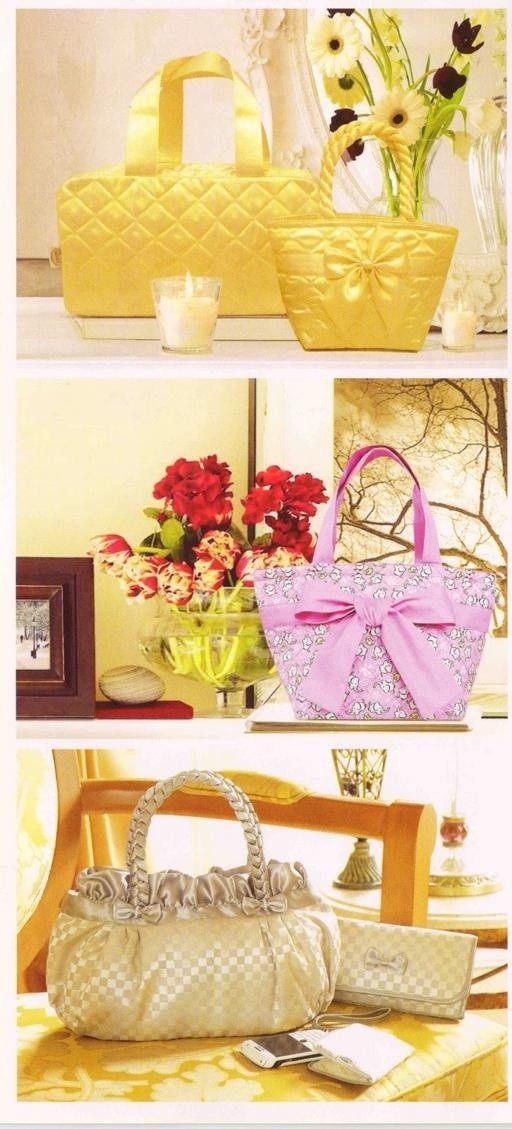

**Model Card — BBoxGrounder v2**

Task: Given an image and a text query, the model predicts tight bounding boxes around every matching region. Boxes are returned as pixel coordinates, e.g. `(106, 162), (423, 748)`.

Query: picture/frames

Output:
(15, 558), (96, 719)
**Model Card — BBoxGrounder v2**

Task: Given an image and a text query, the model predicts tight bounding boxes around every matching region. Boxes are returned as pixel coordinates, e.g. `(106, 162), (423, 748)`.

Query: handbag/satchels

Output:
(59, 53), (316, 317)
(269, 118), (458, 349)
(46, 769), (341, 1042)
(254, 445), (494, 722)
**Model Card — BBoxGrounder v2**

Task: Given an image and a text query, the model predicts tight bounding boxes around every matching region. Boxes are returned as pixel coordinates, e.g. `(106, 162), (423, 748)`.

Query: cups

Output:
(154, 274), (223, 354)
(439, 281), (492, 352)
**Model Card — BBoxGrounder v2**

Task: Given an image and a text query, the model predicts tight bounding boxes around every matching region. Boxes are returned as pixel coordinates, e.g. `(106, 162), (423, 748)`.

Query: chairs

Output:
(18, 749), (504, 1103)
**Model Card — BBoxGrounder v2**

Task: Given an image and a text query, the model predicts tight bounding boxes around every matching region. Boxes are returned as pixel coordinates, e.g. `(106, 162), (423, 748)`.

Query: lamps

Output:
(329, 747), (385, 890)
(416, 747), (503, 897)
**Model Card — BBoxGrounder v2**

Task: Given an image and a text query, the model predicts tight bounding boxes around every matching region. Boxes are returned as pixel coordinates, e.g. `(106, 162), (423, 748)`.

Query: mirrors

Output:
(237, 9), (508, 261)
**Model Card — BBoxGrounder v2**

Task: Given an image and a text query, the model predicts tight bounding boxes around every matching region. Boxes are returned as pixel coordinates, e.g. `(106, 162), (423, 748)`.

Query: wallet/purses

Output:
(333, 917), (477, 1019)
(308, 1021), (414, 1086)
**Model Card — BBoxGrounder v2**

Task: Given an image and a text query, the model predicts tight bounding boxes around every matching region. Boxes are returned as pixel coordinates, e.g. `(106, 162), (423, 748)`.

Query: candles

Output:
(161, 274), (215, 346)
(443, 300), (475, 351)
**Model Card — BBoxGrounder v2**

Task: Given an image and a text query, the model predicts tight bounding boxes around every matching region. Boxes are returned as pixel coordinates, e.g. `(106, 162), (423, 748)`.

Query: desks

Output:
(312, 850), (507, 951)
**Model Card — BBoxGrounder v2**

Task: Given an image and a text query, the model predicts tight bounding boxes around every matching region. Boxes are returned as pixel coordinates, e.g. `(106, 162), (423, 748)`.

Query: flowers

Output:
(88, 454), (331, 602)
(308, 9), (503, 165)
(473, 11), (508, 250)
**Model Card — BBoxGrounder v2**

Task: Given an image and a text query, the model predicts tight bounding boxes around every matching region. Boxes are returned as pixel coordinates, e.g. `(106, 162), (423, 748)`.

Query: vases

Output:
(369, 140), (446, 223)
(136, 587), (275, 716)
(469, 97), (507, 263)
